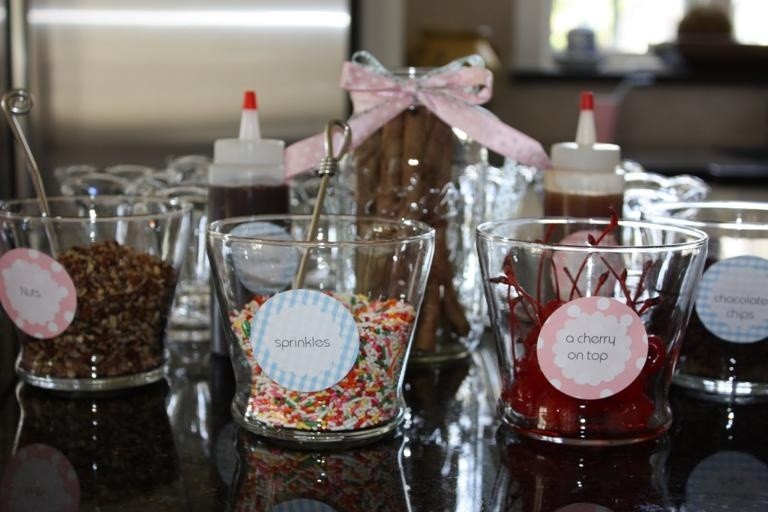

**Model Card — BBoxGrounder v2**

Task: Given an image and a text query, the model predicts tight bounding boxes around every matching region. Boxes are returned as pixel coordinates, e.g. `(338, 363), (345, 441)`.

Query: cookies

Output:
(355, 104), (471, 355)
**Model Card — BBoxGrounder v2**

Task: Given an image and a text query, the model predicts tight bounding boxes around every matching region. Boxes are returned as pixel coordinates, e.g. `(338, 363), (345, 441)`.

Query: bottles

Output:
(351, 64), (487, 365)
(544, 89), (622, 307)
(207, 89), (287, 358)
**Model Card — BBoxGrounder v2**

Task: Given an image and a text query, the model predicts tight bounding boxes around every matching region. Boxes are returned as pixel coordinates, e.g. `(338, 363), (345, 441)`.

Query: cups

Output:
(655, 200), (768, 396)
(476, 220), (709, 445)
(1, 199), (194, 393)
(206, 215), (436, 445)
(460, 155), (708, 218)
(158, 198), (214, 374)
(62, 154), (211, 195)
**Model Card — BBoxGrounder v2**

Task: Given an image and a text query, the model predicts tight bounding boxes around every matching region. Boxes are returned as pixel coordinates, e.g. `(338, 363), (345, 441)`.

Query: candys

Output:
(227, 293), (417, 431)
